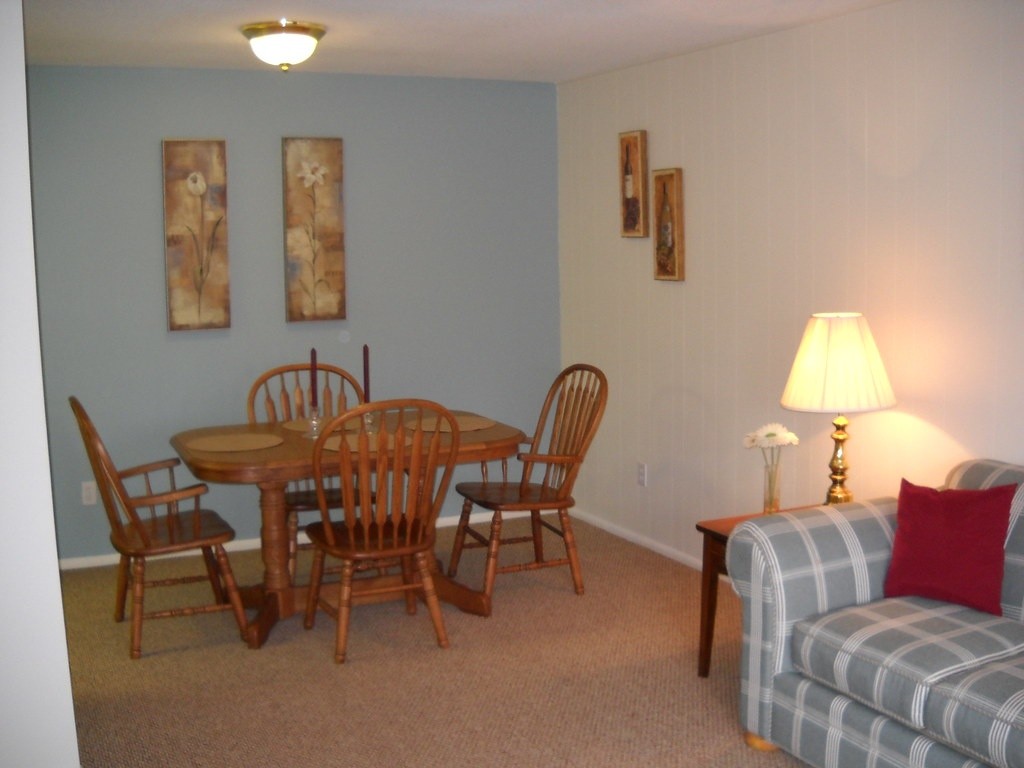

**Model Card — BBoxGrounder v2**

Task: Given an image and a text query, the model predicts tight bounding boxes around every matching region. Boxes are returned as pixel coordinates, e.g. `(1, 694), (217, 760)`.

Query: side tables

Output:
(695, 501), (825, 679)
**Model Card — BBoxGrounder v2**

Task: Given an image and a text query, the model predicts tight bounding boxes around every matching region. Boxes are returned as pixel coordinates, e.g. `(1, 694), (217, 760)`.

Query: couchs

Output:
(727, 456), (1023, 768)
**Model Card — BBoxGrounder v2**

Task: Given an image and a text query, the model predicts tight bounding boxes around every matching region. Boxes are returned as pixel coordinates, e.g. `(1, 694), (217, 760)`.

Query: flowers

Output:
(743, 422), (800, 509)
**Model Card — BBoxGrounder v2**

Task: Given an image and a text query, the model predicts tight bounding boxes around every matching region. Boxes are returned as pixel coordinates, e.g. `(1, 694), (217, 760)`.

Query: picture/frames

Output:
(617, 130), (649, 238)
(651, 167), (685, 281)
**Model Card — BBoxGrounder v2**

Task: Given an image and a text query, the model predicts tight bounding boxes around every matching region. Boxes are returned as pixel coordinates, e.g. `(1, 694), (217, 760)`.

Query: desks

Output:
(169, 408), (528, 651)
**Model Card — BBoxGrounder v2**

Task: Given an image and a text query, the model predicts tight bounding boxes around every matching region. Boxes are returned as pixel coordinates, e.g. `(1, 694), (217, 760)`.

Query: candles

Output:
(310, 347), (318, 412)
(362, 344), (370, 404)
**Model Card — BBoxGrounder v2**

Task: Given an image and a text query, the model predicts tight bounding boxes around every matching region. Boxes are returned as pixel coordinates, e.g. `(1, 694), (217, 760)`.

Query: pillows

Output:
(882, 476), (1019, 616)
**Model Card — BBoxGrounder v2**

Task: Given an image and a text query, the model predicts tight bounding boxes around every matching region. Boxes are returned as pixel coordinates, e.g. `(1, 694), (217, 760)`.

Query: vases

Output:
(762, 463), (781, 518)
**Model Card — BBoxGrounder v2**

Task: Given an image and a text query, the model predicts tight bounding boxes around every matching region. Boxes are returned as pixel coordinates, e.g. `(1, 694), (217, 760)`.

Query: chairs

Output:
(304, 398), (460, 664)
(68, 395), (247, 661)
(246, 363), (376, 590)
(447, 364), (608, 602)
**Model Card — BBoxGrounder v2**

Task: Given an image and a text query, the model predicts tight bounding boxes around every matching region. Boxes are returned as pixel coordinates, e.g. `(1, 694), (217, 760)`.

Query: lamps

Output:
(780, 313), (898, 507)
(239, 22), (327, 73)
